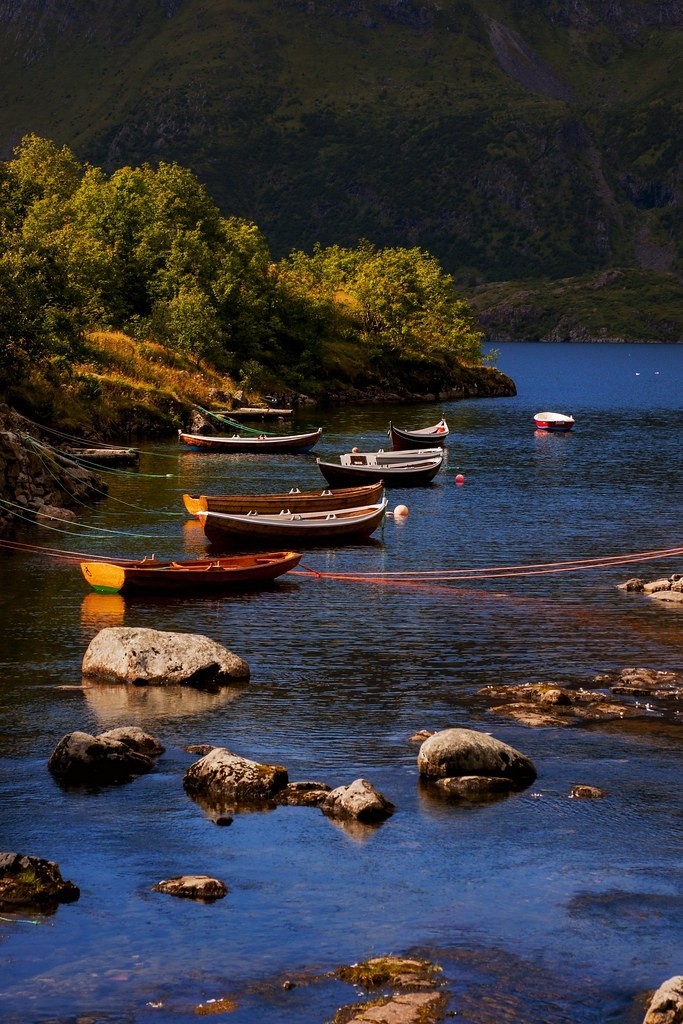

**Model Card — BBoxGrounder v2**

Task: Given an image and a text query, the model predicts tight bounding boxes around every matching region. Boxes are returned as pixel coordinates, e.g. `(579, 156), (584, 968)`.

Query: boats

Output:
(184, 477), (386, 523)
(315, 445), (443, 487)
(200, 499), (388, 544)
(534, 411), (576, 433)
(176, 425), (324, 454)
(388, 417), (449, 449)
(74, 548), (303, 592)
